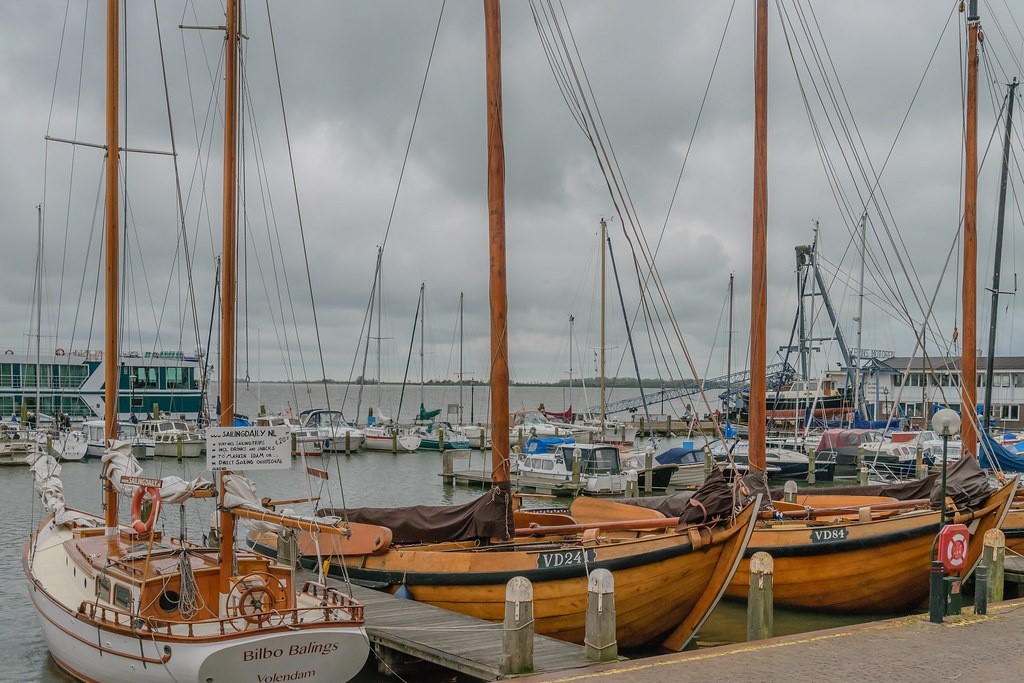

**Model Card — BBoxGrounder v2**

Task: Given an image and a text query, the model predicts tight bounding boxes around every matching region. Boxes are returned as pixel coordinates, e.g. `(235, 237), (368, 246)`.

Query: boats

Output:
(726, 234), (860, 429)
(0, 350), (209, 422)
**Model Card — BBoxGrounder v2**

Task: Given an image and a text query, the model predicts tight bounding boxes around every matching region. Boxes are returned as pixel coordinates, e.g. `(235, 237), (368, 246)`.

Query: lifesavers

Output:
(130, 484), (161, 534)
(4, 349), (14, 356)
(54, 348), (65, 357)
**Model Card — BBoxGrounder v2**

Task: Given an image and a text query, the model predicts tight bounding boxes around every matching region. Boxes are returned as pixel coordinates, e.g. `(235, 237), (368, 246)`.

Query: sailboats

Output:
(0, 191), (643, 465)
(25, 0), (1024, 683)
